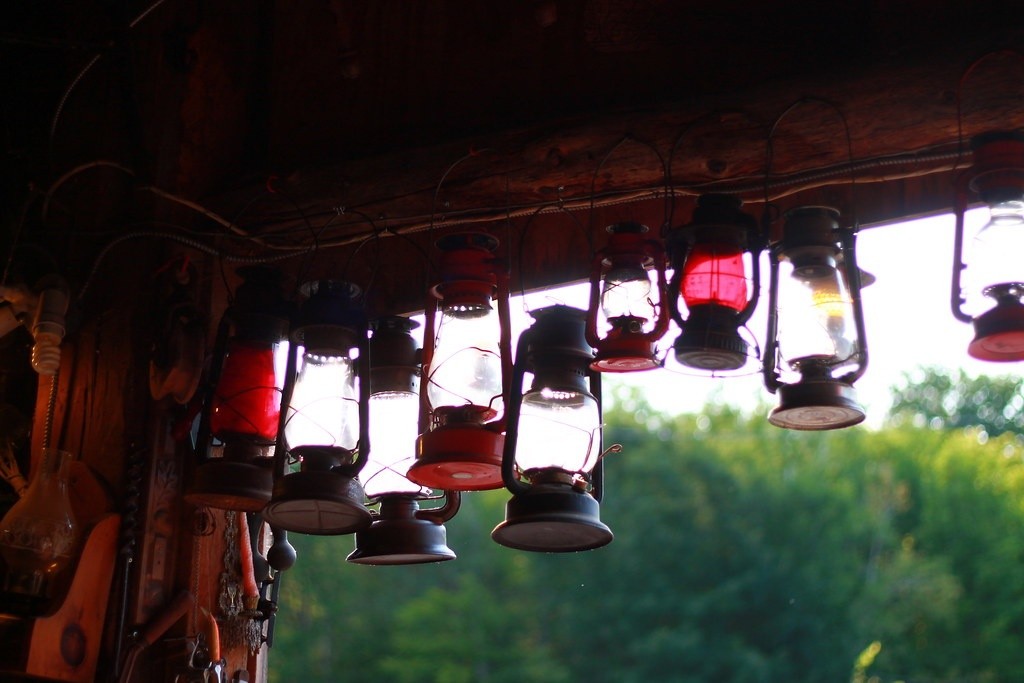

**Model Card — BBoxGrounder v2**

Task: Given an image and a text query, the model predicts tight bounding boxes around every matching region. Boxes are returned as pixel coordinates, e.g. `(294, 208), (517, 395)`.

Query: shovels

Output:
(118, 590), (194, 683)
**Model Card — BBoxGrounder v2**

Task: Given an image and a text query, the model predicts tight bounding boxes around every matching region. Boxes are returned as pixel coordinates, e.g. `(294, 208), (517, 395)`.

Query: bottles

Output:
(0, 446), (84, 620)
